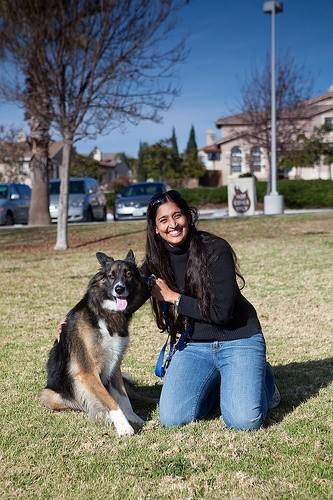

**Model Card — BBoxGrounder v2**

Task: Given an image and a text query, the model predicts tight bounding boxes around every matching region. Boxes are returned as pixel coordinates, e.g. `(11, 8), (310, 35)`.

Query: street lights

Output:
(264, 0), (284, 214)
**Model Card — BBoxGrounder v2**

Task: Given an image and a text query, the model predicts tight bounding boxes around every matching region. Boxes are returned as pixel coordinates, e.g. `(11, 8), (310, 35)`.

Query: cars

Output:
(115, 183), (166, 220)
(1, 183), (32, 225)
(48, 177), (107, 223)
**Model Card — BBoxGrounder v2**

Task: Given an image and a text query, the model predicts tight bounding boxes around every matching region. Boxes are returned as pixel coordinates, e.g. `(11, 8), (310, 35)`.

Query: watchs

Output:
(174, 294), (182, 307)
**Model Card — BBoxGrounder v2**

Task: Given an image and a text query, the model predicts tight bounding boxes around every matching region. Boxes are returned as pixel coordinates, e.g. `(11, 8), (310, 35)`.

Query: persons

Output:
(55, 190), (283, 430)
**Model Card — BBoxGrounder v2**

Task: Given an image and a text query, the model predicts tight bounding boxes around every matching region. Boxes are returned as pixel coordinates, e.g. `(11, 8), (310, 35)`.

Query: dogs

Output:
(39, 249), (149, 439)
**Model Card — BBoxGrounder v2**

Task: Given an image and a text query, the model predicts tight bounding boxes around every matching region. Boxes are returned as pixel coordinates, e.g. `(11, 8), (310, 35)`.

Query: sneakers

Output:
(268, 383), (281, 411)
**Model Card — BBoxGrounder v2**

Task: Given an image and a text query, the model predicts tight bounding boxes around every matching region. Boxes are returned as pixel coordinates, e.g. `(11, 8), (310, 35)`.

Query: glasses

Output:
(150, 190), (181, 207)
